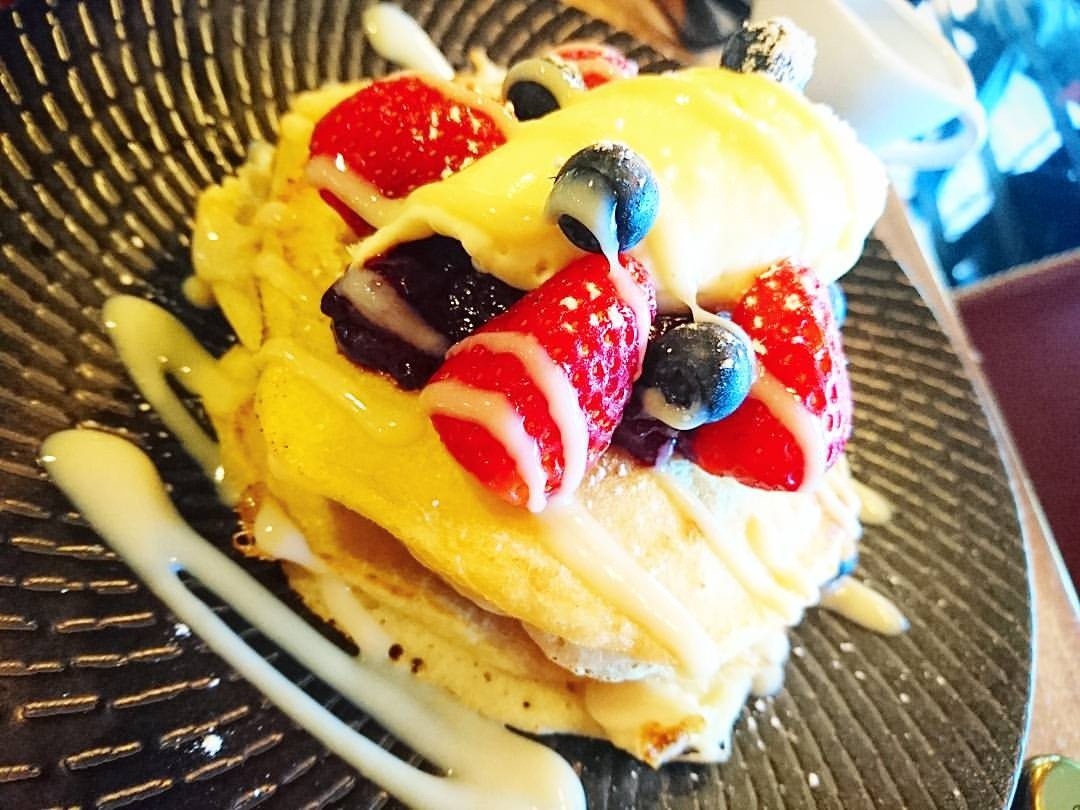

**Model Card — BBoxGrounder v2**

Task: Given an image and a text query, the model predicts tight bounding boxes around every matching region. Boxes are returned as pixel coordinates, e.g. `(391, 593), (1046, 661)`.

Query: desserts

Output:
(188, 12), (903, 769)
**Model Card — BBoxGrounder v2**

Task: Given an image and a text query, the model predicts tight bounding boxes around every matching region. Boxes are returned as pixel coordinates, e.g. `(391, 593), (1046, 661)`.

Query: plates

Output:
(1, 0), (1033, 809)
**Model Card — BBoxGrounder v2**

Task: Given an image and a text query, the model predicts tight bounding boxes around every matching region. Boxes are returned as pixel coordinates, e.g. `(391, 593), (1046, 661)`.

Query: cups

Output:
(744, 0), (987, 173)
